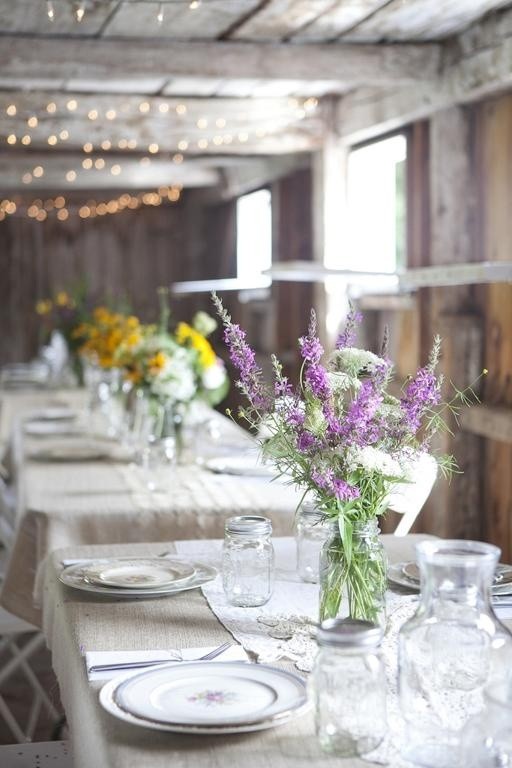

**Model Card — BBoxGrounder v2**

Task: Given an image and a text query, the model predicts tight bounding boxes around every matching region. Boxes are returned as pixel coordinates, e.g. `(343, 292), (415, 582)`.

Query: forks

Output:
(87, 641), (235, 674)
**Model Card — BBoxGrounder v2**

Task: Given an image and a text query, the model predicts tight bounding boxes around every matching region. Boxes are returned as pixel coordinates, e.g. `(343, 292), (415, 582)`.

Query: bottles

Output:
(396, 538), (511, 768)
(144, 433), (177, 498)
(295, 496), (332, 585)
(311, 616), (389, 759)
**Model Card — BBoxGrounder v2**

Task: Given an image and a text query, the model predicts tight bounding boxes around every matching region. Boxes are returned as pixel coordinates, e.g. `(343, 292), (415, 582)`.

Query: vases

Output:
(318, 523), (389, 634)
(296, 511), (324, 585)
(305, 519), (394, 635)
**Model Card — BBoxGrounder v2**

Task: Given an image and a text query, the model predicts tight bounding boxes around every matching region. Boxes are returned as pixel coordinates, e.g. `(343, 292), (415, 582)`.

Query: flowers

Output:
(218, 297), (491, 603)
(73, 307), (218, 402)
(211, 291), (440, 499)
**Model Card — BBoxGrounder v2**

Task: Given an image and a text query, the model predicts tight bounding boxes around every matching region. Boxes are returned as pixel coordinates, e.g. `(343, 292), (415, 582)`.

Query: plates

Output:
(83, 557), (197, 590)
(403, 560), (512, 588)
(387, 560), (510, 592)
(21, 406), (123, 462)
(59, 557), (218, 604)
(114, 661), (309, 726)
(95, 662), (320, 737)
(207, 455), (283, 476)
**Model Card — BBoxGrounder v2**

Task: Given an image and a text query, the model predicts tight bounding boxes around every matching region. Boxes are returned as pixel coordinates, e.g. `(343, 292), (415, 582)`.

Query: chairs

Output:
(4, 736), (74, 766)
(0, 606), (62, 744)
